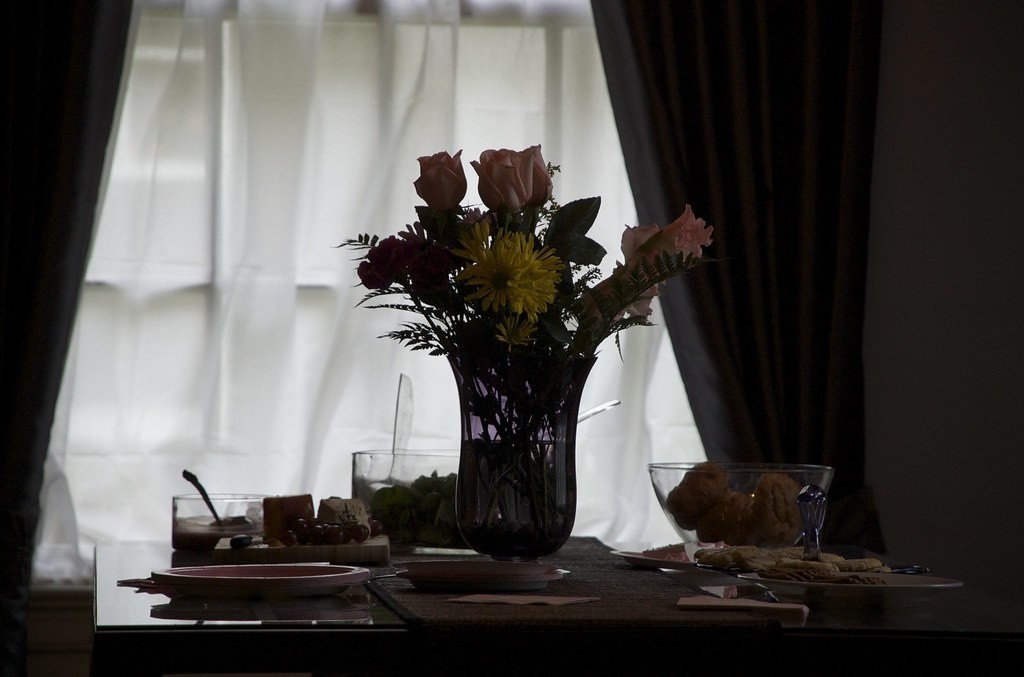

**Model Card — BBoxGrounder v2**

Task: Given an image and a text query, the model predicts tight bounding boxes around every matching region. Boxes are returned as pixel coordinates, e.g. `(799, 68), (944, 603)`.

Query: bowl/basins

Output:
(351, 448), (486, 557)
(647, 461), (835, 563)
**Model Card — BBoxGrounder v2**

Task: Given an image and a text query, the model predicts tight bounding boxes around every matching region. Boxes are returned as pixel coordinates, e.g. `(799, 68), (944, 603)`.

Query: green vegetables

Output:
(369, 472), (467, 550)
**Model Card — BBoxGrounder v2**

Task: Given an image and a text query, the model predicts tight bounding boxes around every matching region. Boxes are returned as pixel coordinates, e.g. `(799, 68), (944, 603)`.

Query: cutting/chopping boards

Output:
(212, 533), (391, 566)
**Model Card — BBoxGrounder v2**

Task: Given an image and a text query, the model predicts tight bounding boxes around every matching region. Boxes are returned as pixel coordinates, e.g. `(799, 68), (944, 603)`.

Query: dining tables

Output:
(91, 538), (1024, 677)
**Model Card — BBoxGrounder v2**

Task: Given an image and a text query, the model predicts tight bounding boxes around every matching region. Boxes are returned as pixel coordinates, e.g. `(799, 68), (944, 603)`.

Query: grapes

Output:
(275, 513), (382, 546)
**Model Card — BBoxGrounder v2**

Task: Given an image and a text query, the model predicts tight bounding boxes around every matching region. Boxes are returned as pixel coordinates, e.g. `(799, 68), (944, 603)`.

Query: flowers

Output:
(340, 144), (717, 536)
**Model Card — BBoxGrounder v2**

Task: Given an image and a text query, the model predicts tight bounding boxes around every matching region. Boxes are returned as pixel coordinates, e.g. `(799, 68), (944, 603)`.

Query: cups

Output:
(171, 493), (280, 551)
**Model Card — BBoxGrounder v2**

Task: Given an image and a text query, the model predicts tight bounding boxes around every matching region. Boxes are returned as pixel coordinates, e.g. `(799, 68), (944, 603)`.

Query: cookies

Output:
(694, 545), (884, 574)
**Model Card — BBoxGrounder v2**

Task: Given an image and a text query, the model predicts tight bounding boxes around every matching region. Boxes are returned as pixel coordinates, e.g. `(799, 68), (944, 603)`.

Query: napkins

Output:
(677, 595), (809, 617)
(453, 592), (603, 609)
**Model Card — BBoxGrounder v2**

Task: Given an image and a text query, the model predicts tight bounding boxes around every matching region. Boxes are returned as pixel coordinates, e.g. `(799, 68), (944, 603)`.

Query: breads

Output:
(666, 462), (803, 548)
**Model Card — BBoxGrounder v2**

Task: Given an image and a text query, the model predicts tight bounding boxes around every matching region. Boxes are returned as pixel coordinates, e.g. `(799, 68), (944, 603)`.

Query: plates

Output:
(737, 572), (964, 611)
(392, 560), (571, 593)
(693, 560), (935, 580)
(150, 562), (372, 600)
(609, 548), (696, 570)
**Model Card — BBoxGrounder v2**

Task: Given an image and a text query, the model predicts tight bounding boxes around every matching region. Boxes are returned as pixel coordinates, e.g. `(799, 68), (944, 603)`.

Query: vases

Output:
(447, 349), (596, 560)
